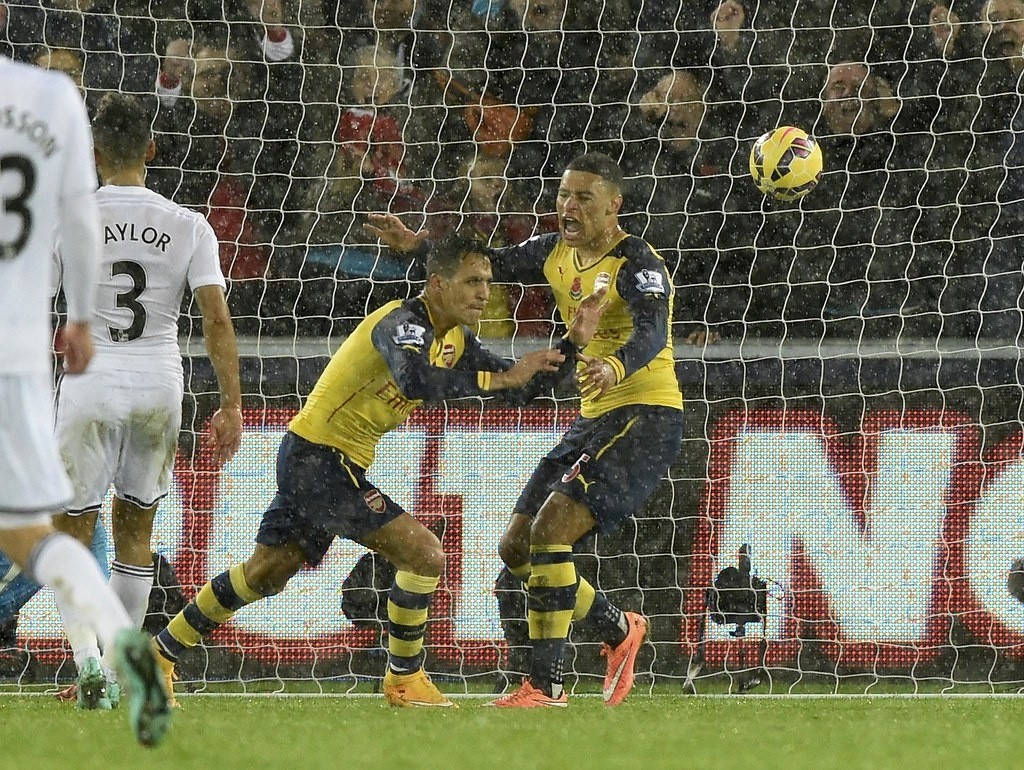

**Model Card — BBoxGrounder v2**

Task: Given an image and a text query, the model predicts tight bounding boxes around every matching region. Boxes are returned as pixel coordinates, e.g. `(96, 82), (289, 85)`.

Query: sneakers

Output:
(151, 635), (181, 707)
(54, 680), (120, 709)
(76, 657), (112, 710)
(115, 629), (172, 749)
(481, 676), (567, 708)
(599, 611), (647, 706)
(383, 666), (459, 707)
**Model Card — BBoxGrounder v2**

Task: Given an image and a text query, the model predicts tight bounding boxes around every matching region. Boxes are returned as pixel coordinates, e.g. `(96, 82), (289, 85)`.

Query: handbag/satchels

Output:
(434, 69), (533, 156)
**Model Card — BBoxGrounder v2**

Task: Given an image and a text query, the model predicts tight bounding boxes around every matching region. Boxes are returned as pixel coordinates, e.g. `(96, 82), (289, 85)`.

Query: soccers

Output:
(748, 125), (824, 201)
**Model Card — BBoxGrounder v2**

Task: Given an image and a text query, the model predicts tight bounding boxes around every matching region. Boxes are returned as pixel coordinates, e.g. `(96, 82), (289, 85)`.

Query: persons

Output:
(0, 0), (1024, 339)
(53, 89), (244, 713)
(1, 4), (171, 749)
(1, 510), (112, 704)
(362, 152), (685, 711)
(148, 232), (611, 711)
